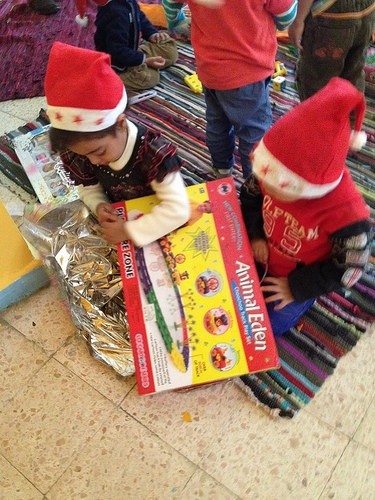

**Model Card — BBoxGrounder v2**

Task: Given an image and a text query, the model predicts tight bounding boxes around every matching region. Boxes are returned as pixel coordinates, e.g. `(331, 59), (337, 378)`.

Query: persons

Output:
(238, 76), (370, 332)
(160, 0), (298, 181)
(94, 0), (180, 90)
(287, 0), (375, 102)
(44, 41), (190, 246)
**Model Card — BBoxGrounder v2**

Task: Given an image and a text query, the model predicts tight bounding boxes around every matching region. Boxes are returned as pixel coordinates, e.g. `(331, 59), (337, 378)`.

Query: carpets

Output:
(0, 39), (375, 422)
(0, 0), (163, 102)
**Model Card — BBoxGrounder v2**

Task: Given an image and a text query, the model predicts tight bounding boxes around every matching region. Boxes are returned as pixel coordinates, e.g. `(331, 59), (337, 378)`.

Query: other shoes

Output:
(212, 168), (234, 179)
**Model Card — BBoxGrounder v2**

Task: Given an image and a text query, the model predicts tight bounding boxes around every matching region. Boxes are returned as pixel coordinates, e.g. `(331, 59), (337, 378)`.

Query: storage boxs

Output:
(11, 124), (80, 205)
(110, 176), (281, 397)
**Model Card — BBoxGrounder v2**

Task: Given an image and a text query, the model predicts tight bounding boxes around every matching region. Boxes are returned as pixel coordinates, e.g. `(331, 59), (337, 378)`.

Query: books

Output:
(111, 175), (280, 397)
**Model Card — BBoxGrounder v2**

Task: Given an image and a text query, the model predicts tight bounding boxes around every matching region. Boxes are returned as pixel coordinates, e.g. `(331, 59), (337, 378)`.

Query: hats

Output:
(44, 40), (128, 133)
(250, 76), (368, 199)
